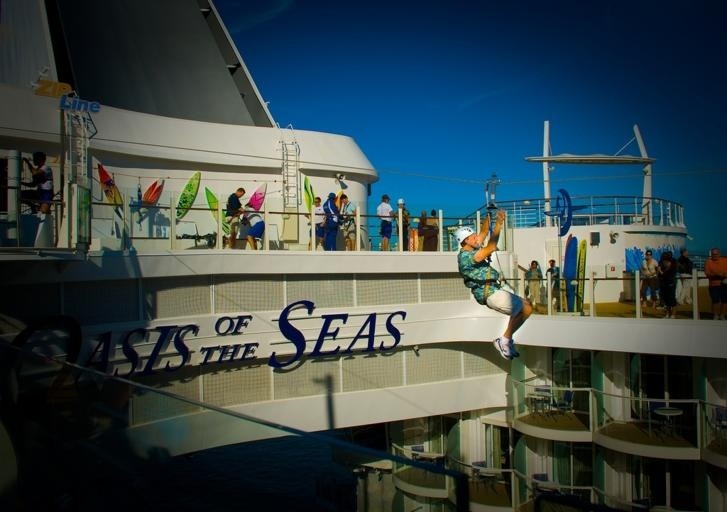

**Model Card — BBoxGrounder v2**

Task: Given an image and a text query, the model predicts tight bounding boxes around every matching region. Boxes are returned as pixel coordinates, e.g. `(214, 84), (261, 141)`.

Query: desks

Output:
(654, 406), (683, 441)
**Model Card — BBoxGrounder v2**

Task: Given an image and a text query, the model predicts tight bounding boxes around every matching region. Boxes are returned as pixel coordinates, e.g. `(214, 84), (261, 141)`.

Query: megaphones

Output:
(336, 173), (346, 182)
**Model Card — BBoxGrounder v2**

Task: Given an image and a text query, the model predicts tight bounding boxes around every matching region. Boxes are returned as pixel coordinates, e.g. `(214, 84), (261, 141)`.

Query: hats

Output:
(398, 198), (405, 204)
(454, 226), (475, 244)
(383, 195), (391, 200)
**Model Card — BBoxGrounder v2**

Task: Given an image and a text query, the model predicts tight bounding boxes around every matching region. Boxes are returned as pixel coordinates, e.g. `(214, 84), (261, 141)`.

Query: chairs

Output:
(526, 385), (574, 424)
(407, 444), (653, 511)
(713, 406), (726, 441)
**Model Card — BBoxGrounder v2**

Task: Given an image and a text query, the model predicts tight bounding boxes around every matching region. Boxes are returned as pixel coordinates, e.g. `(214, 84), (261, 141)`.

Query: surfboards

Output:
(133, 178), (164, 224)
(240, 184), (266, 228)
(304, 175), (315, 219)
(205, 187), (229, 235)
(334, 191), (344, 215)
(410, 227), (419, 253)
(176, 172), (200, 224)
(97, 162), (125, 221)
(563, 233), (587, 314)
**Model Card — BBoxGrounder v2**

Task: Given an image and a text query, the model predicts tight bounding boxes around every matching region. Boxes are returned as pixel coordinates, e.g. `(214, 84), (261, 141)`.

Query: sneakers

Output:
(670, 314), (676, 319)
(712, 314), (720, 321)
(663, 314), (669, 319)
(719, 314), (726, 320)
(493, 336), (521, 360)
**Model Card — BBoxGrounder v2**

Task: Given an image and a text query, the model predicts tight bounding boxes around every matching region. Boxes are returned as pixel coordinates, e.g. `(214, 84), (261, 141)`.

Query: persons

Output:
(224, 187), (246, 248)
(17, 153), (54, 218)
(639, 247), (696, 318)
(425, 209), (438, 249)
(320, 192), (344, 253)
(338, 194), (359, 251)
(376, 194), (393, 251)
(417, 210), (426, 238)
(240, 203), (265, 251)
(545, 260), (562, 309)
(704, 248), (726, 321)
(305, 197), (327, 252)
(452, 208), (532, 361)
(524, 260), (543, 309)
(394, 198), (410, 250)
